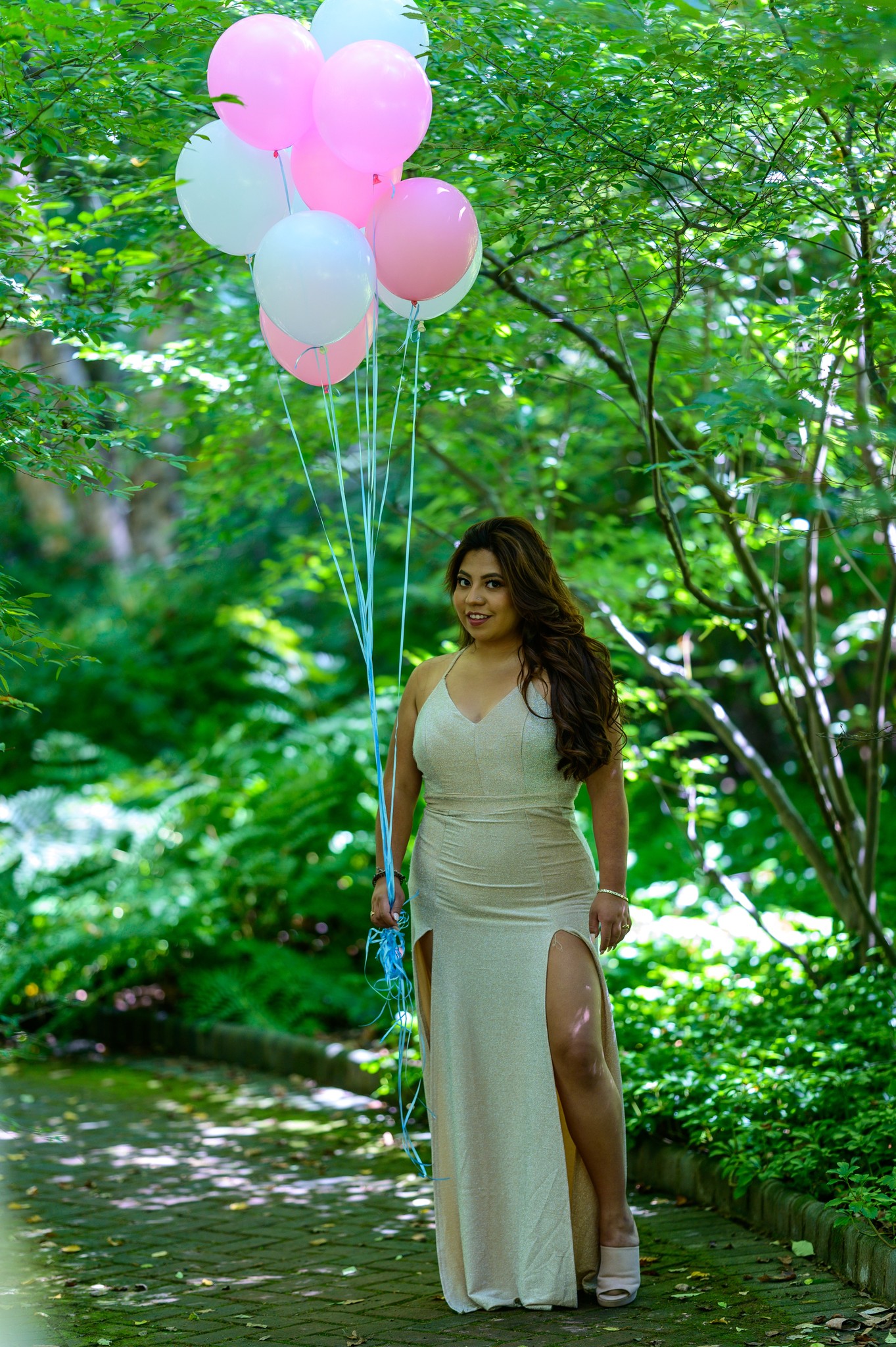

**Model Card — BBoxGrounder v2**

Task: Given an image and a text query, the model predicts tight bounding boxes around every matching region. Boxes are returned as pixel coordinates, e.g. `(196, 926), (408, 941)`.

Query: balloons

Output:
(207, 13), (324, 158)
(254, 210), (376, 354)
(258, 294), (379, 393)
(312, 39), (432, 185)
(175, 118), (294, 264)
(285, 145), (366, 236)
(309, 0), (429, 71)
(293, 125), (404, 231)
(375, 230), (483, 332)
(365, 176), (479, 308)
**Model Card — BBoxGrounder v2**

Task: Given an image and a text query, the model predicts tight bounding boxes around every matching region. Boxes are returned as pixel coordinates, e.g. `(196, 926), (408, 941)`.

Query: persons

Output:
(371, 516), (641, 1310)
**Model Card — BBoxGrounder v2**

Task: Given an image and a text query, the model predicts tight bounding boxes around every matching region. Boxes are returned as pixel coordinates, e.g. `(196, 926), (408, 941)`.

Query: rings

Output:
(370, 911), (374, 916)
(621, 924), (630, 929)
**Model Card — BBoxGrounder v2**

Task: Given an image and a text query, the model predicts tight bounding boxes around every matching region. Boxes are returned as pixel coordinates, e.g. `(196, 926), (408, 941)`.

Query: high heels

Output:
(596, 1244), (641, 1308)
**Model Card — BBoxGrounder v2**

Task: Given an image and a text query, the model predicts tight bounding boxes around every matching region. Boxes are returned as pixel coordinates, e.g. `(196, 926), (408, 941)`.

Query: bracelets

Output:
(372, 866), (406, 887)
(597, 889), (629, 902)
